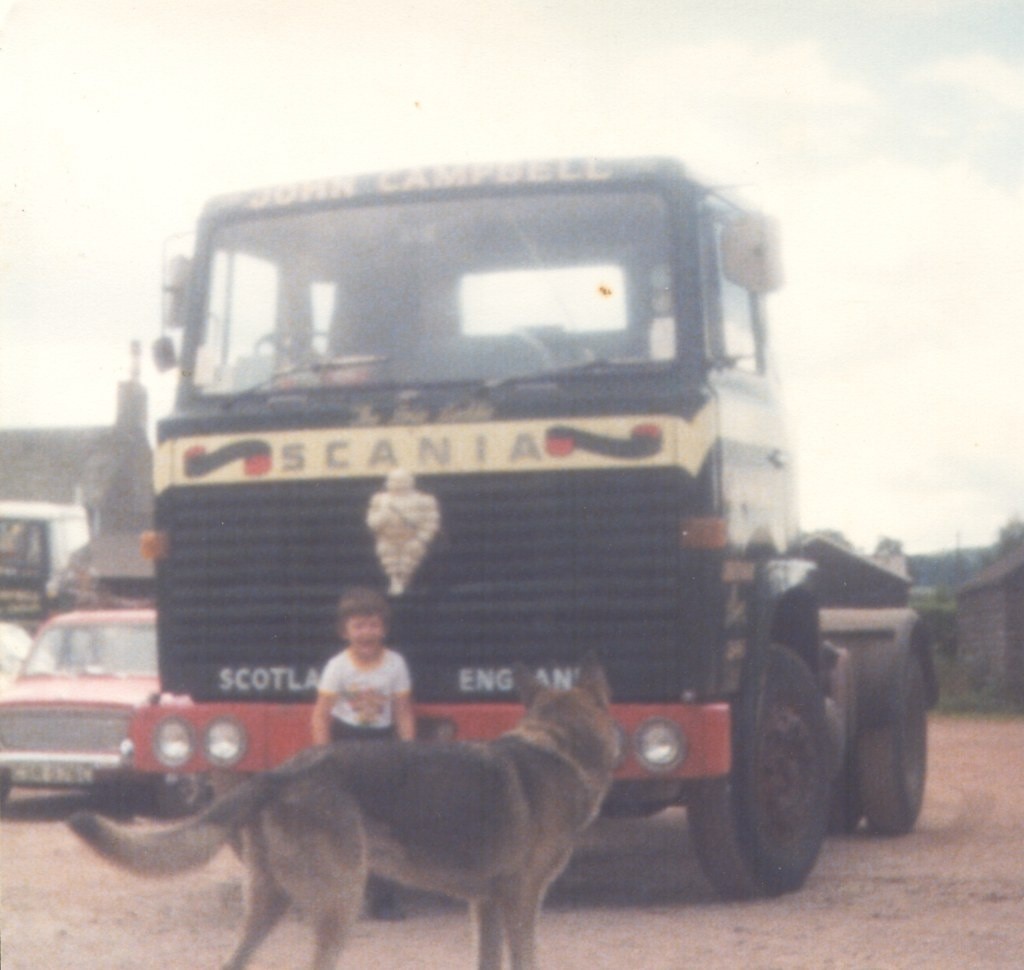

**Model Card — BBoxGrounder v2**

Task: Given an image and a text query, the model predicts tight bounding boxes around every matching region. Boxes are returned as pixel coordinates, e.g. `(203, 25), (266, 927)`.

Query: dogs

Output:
(54, 646), (625, 970)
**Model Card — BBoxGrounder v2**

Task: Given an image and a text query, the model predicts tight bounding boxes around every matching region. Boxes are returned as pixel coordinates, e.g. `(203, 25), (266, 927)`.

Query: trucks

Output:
(118, 155), (927, 899)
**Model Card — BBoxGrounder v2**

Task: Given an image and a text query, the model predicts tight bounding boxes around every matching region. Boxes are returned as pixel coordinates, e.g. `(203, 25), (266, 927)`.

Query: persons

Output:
(309, 586), (417, 921)
(365, 465), (442, 595)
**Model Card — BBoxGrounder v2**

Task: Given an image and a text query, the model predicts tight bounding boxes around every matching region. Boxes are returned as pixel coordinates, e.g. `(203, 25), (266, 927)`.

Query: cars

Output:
(1, 606), (206, 823)
